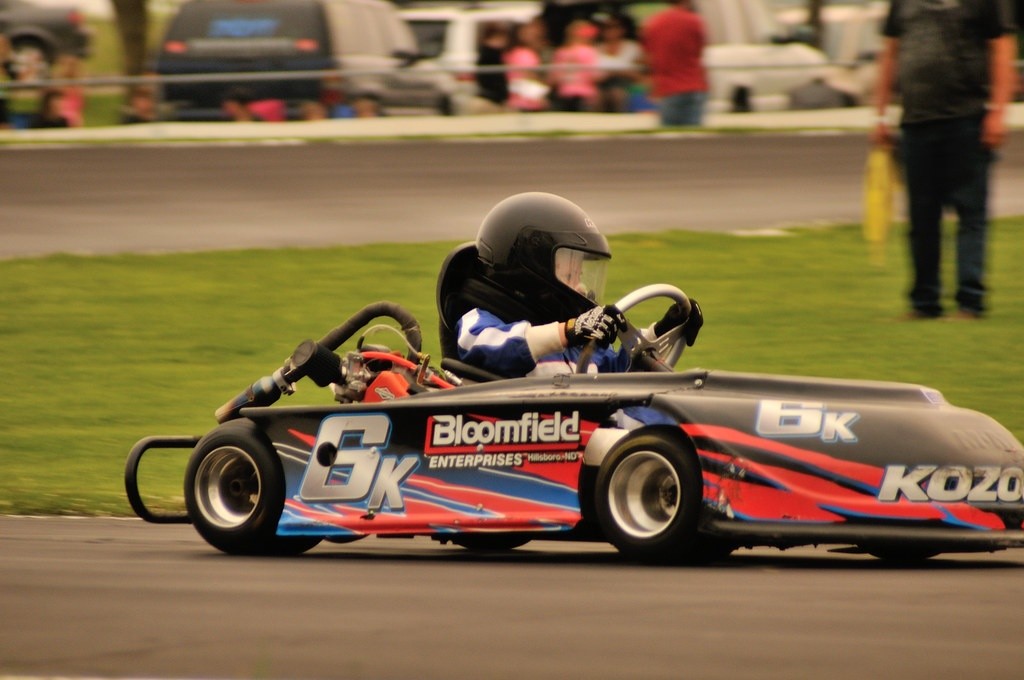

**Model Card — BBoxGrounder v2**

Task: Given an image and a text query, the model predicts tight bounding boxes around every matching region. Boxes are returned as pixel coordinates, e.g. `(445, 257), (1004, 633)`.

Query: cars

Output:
(0, 1), (899, 129)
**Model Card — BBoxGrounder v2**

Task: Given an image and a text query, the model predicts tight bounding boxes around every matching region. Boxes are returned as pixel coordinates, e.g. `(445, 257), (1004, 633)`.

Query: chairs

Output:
(437, 241), (478, 360)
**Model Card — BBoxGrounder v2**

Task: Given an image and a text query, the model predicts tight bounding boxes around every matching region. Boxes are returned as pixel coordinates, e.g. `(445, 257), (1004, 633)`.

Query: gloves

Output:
(654, 296), (703, 347)
(562, 304), (627, 350)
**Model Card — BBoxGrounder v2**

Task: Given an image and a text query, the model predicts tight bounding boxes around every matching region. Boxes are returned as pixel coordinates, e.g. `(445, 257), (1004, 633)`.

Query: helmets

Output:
(476, 191), (611, 321)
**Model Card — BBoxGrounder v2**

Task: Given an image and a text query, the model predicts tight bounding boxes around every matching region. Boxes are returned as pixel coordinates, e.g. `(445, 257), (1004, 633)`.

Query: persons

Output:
(870, 0), (1013, 320)
(443, 192), (704, 379)
(0, 0), (884, 130)
